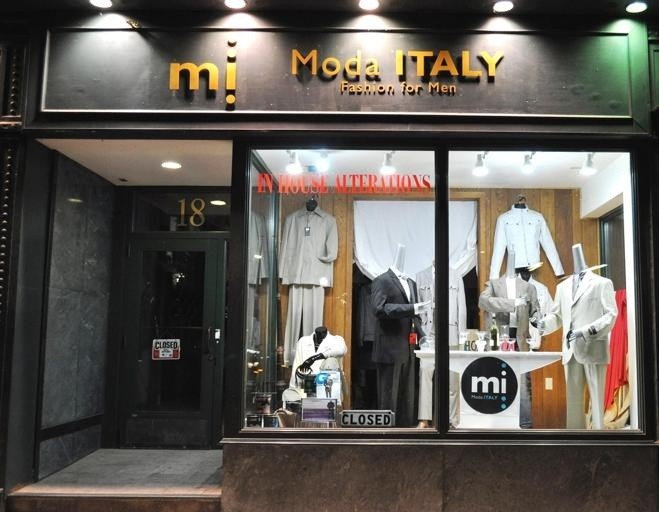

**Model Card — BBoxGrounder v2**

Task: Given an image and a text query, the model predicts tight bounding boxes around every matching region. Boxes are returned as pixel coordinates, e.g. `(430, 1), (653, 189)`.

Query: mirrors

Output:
(244, 149), (280, 427)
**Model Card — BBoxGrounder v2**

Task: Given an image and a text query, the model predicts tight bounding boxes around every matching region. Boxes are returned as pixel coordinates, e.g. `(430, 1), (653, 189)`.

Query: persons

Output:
(490, 202), (562, 287)
(282, 200), (337, 365)
(535, 270), (619, 430)
(476, 273), (543, 429)
(371, 265), (433, 427)
(415, 258), (467, 429)
(290, 325), (349, 412)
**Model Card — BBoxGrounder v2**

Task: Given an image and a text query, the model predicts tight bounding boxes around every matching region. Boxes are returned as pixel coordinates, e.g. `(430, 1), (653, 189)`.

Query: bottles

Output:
(489, 312), (500, 351)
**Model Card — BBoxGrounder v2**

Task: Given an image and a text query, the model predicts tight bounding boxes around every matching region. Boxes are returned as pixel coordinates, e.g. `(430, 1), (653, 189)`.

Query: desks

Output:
(414, 350), (564, 429)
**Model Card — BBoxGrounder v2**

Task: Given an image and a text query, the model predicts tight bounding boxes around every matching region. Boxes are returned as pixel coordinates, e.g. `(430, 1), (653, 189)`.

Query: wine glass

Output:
(506, 338), (517, 351)
(526, 339), (538, 352)
